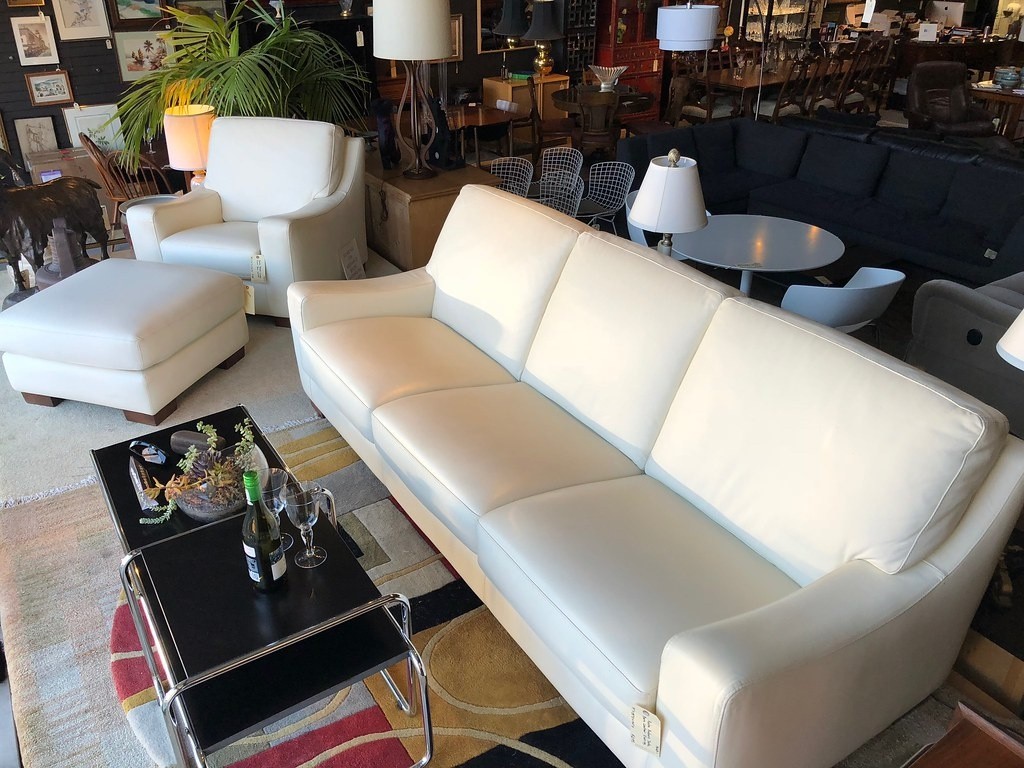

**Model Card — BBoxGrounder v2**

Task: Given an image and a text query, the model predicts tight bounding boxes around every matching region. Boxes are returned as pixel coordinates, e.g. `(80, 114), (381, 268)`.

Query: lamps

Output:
(655, 1), (720, 53)
(163, 104), (218, 192)
(521, 4), (565, 77)
(373, 2), (453, 180)
(494, 1), (528, 49)
(631, 148), (707, 263)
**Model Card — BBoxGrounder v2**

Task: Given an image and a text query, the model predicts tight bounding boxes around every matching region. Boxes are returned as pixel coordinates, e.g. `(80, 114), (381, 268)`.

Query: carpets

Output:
(0, 412), (623, 768)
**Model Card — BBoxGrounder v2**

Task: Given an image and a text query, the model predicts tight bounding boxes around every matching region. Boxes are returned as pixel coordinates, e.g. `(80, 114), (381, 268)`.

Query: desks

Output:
(670, 213), (846, 296)
(119, 147), (191, 194)
(551, 85), (653, 149)
(964, 79), (1024, 140)
(690, 55), (890, 117)
(481, 75), (570, 161)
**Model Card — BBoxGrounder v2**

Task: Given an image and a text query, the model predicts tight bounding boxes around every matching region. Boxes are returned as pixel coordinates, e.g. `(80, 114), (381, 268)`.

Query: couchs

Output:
(124, 114), (368, 325)
(286, 180), (1023, 767)
(904, 62), (1000, 133)
(904, 271), (1023, 436)
(616, 118), (1024, 295)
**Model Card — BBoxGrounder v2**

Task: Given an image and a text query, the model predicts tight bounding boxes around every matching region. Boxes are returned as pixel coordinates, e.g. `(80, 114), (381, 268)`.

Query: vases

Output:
(615, 30), (624, 42)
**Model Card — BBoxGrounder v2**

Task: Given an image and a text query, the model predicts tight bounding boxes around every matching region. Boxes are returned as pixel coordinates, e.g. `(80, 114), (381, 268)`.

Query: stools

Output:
(945, 133), (1021, 161)
(0, 259), (251, 428)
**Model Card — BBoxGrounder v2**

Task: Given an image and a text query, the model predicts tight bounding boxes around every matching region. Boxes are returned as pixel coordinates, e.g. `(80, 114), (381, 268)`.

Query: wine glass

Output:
(143, 128), (156, 154)
(745, 0), (806, 73)
(736, 47), (745, 81)
(279, 480), (328, 569)
(251, 472), (293, 551)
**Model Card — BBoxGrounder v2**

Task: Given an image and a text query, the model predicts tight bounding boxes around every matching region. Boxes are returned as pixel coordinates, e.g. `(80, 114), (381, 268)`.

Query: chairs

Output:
(505, 78), (563, 161)
(78, 133), (157, 250)
(107, 148), (173, 205)
(574, 92), (623, 160)
(487, 130), (712, 244)
(672, 26), (898, 117)
(780, 266), (906, 335)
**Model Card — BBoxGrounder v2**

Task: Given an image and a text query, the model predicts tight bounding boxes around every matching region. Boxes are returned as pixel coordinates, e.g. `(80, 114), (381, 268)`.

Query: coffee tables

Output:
(118, 512), (434, 768)
(87, 407), (302, 554)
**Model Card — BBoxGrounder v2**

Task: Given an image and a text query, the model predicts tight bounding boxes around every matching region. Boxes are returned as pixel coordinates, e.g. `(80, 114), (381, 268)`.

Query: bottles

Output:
(501, 52), (509, 79)
(243, 468), (287, 596)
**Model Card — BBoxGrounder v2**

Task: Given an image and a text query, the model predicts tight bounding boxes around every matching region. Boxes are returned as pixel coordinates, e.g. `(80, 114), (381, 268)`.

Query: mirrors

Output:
(477, 0), (537, 55)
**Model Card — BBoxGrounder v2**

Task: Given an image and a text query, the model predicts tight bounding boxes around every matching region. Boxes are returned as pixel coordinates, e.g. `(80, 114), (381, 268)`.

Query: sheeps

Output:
(0, 148), (109, 291)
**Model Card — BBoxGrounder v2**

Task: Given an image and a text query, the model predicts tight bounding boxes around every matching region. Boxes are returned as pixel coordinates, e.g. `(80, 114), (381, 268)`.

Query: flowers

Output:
(606, 22), (629, 33)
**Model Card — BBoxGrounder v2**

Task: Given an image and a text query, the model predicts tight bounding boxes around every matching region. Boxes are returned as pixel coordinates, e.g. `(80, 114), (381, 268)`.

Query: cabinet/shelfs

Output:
(601, 0), (667, 121)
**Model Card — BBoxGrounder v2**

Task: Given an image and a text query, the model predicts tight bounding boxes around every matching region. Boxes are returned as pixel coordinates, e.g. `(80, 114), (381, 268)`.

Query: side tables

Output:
(362, 165), (499, 272)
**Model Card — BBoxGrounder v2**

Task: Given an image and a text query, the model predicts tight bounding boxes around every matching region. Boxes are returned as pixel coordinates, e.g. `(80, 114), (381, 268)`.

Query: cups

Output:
(339, 0), (353, 16)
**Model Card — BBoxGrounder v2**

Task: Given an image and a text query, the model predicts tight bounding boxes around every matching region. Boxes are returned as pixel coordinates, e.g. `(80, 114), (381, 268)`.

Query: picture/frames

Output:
(111, 30), (180, 86)
(15, 118), (58, 172)
(6, 0), (45, 7)
(9, 11), (63, 65)
(47, 0), (110, 44)
(25, 71), (75, 107)
(421, 15), (462, 64)
(106, 0), (170, 31)
(61, 105), (127, 152)
(173, 0), (228, 39)
(0, 116), (11, 155)
